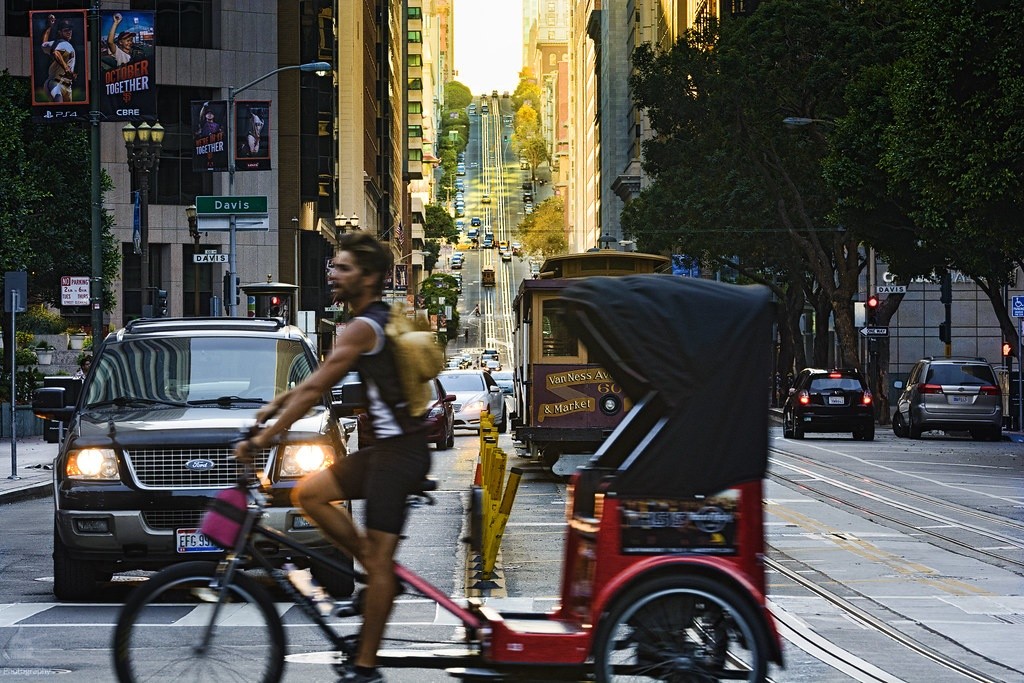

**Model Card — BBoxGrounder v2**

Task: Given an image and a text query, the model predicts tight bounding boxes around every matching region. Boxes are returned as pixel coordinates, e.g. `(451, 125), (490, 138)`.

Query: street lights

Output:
(122, 120), (166, 317)
(229, 61), (331, 315)
(185, 205), (202, 316)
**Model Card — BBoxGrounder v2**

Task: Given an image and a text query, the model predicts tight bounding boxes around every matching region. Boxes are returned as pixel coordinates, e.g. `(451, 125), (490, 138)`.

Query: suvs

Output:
(784, 368), (875, 442)
(51, 315), (348, 603)
(893, 355), (1004, 442)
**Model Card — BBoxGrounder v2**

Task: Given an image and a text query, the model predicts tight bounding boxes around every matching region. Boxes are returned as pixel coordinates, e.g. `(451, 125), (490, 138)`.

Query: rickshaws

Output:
(108, 274), (787, 683)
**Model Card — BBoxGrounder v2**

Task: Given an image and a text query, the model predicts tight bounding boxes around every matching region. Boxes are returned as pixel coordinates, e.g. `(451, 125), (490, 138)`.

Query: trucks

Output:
(482, 265), (496, 287)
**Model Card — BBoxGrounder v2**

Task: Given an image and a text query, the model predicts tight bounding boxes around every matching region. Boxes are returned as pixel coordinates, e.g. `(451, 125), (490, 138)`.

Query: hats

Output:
(383, 301), (444, 416)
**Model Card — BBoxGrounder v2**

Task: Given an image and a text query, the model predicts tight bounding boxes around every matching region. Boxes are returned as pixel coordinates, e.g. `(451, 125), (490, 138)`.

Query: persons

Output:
(107, 15), (140, 66)
(75, 359), (91, 383)
(235, 232), (433, 683)
(195, 102), (266, 168)
(41, 13), (79, 103)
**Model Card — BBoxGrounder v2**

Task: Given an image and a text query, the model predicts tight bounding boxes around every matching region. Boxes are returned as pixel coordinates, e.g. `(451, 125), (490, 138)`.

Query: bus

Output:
(514, 248), (673, 461)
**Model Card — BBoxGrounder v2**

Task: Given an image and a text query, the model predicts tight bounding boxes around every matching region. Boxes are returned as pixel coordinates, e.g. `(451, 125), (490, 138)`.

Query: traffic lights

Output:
(867, 297), (880, 329)
(269, 295), (282, 314)
(156, 290), (167, 314)
(1002, 341), (1016, 359)
(225, 274), (241, 306)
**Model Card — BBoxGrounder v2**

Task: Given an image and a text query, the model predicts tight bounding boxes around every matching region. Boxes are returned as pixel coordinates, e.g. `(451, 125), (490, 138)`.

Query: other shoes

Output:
(337, 667), (383, 683)
(329, 581), (406, 617)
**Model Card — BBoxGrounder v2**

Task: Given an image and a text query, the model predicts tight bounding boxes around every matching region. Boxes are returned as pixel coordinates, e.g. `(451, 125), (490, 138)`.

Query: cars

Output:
(454, 179), (465, 192)
(521, 180), (534, 217)
(467, 228), (479, 242)
(454, 192), (465, 218)
(403, 367), (524, 449)
(470, 216), (481, 225)
(447, 272), (463, 293)
(479, 350), (502, 372)
(482, 232), (496, 248)
(454, 221), (465, 234)
(451, 252), (465, 269)
(481, 195), (492, 203)
(498, 239), (522, 261)
(454, 153), (466, 175)
